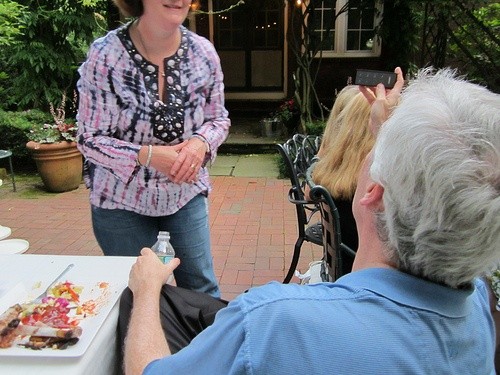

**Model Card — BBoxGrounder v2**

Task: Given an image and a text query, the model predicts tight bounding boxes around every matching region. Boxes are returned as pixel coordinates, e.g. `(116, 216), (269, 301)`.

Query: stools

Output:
(0, 149), (16, 191)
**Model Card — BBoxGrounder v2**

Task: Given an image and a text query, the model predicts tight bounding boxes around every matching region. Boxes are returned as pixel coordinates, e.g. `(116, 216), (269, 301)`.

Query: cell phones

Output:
(351, 69), (397, 89)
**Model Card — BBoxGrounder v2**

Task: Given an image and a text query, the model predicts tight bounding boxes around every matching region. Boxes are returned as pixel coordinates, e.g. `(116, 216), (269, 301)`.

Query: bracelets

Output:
(145, 144), (152, 167)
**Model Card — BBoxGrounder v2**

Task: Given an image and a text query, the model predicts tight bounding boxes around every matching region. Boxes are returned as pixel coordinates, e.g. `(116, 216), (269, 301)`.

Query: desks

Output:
(0, 250), (174, 375)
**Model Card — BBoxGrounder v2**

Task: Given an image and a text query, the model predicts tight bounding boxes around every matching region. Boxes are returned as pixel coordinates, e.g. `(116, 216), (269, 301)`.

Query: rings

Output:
(191, 165), (195, 169)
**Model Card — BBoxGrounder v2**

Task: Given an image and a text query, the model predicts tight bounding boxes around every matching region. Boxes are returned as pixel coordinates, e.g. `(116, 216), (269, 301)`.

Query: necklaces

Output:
(140, 37), (166, 77)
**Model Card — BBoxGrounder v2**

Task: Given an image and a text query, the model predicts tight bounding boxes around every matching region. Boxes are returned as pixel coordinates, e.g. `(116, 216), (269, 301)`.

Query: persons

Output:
(75, 0), (232, 299)
(303, 84), (401, 282)
(115, 66), (500, 375)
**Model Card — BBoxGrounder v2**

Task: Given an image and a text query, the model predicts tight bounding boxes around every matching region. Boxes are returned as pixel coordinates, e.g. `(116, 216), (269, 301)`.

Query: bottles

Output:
(150, 231), (175, 285)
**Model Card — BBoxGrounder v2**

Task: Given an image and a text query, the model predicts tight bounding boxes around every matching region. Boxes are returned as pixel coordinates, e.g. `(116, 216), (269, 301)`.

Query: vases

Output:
(261, 119), (290, 139)
(26, 136), (84, 192)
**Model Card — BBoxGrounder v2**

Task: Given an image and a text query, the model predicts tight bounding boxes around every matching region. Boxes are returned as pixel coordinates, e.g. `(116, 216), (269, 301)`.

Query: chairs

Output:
(273, 133), (357, 285)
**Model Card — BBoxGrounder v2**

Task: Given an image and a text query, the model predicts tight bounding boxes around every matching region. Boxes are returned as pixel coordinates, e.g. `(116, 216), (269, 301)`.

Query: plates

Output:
(0, 273), (129, 357)
(0, 239), (30, 254)
(0, 225), (12, 240)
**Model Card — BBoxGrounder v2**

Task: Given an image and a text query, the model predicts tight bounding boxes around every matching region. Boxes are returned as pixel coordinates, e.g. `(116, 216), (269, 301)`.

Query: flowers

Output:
(26, 92), (86, 145)
(266, 99), (302, 124)
